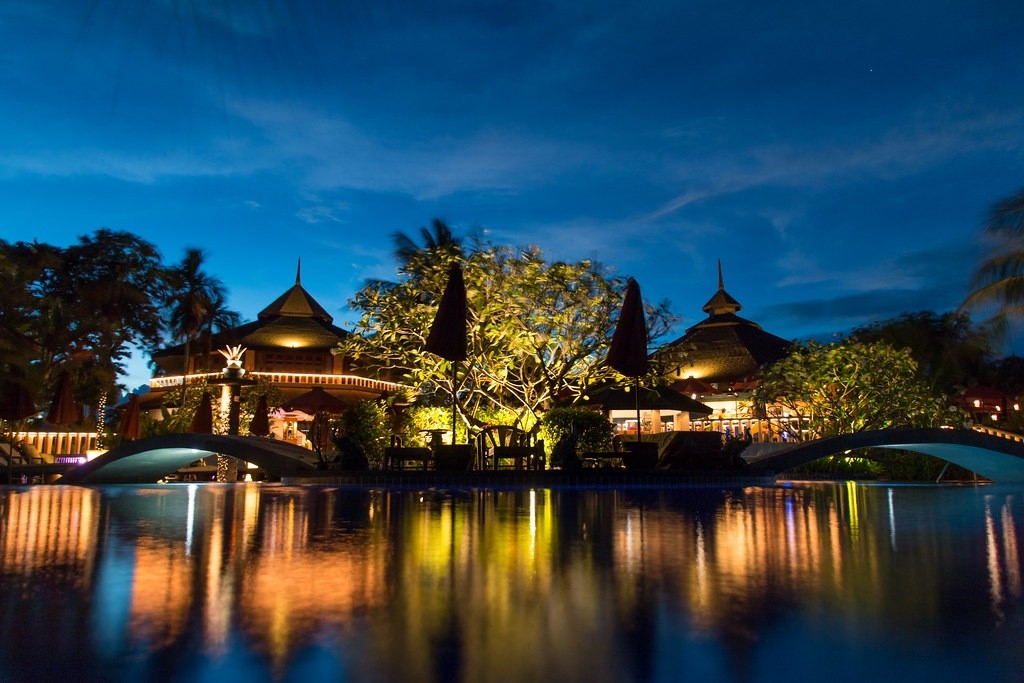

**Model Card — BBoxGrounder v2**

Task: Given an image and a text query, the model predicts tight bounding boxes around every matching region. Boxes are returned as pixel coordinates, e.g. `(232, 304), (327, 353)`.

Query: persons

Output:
(721, 427), (795, 445)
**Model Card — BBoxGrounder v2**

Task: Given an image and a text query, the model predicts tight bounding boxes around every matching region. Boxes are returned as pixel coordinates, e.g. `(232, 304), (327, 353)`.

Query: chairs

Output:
(384, 434), (433, 472)
(475, 425), (542, 472)
(0, 441), (81, 485)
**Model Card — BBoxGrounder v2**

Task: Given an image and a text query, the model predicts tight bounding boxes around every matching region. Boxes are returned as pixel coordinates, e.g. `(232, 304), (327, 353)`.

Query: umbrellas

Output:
(249, 394), (270, 437)
(280, 386), (346, 451)
(115, 393), (140, 445)
(189, 391), (211, 433)
(604, 278), (648, 442)
(421, 262), (467, 446)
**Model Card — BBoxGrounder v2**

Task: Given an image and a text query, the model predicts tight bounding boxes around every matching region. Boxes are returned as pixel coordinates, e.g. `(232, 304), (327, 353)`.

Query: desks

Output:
(434, 444), (475, 471)
(576, 451), (633, 468)
